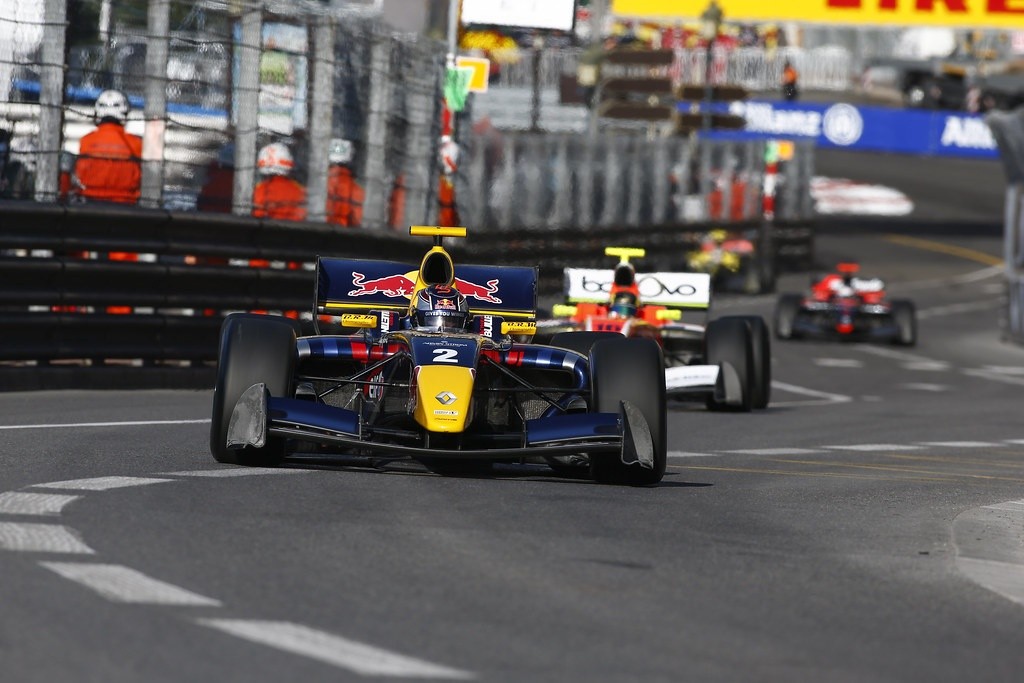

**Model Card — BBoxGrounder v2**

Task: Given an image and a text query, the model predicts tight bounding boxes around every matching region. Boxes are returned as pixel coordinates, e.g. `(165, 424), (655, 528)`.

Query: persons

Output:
(254, 143), (306, 222)
(783, 57), (799, 99)
(326, 139), (364, 226)
(410, 284), (469, 333)
(392, 140), (462, 231)
(610, 290), (639, 318)
(75, 91), (143, 206)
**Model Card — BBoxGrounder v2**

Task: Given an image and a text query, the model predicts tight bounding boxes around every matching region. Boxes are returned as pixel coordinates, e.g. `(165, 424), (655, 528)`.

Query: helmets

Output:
(434, 138), (460, 175)
(93, 89), (129, 127)
(218, 143), (236, 171)
(327, 137), (356, 167)
(609, 291), (639, 318)
(255, 141), (293, 177)
(413, 284), (471, 334)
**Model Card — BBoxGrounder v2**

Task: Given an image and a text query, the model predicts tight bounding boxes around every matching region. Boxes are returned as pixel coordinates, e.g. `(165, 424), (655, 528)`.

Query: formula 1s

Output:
(548, 243), (771, 415)
(206, 223), (670, 486)
(772, 262), (916, 348)
